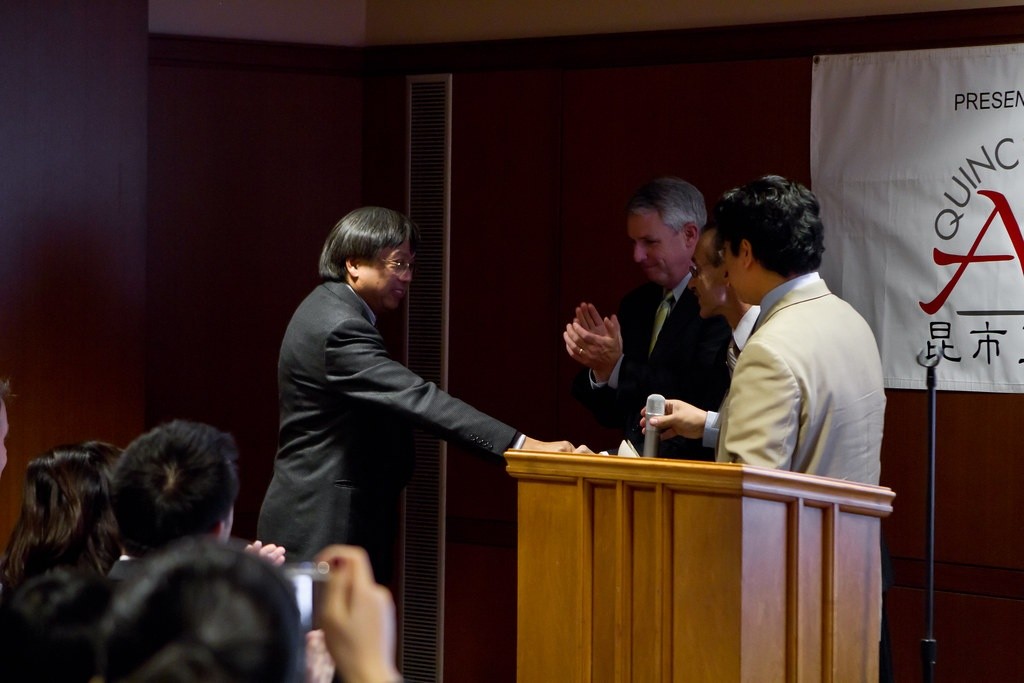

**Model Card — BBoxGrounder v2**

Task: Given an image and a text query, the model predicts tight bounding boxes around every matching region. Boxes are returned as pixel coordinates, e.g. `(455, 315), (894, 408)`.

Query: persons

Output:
(639, 176), (887, 486)
(0, 380), (404, 683)
(255, 206), (575, 560)
(688, 223), (762, 411)
(563, 176), (728, 454)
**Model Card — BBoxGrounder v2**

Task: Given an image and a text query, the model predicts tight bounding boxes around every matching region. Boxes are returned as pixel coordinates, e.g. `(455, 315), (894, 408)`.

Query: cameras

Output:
(280, 566), (332, 634)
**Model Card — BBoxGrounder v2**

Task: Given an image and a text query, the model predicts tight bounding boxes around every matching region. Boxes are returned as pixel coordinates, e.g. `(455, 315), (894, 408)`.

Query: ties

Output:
(726, 337), (737, 378)
(649, 290), (674, 355)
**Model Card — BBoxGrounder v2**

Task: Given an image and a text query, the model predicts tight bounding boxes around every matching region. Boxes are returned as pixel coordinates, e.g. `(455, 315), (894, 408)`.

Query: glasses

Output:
(719, 248), (727, 259)
(689, 259), (717, 278)
(374, 254), (415, 275)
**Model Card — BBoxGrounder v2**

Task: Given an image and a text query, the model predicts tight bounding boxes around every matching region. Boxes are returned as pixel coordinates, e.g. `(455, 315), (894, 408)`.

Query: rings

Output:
(579, 349), (583, 355)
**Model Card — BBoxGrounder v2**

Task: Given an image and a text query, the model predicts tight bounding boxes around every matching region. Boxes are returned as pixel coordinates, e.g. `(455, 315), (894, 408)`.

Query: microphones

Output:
(643, 394), (665, 457)
